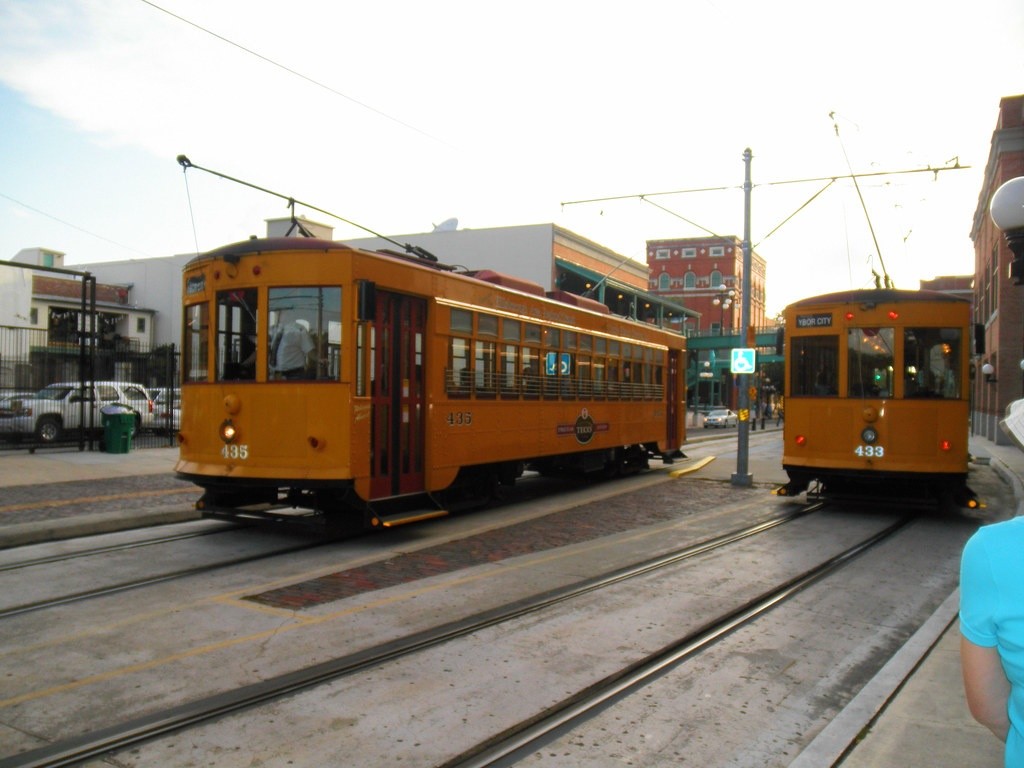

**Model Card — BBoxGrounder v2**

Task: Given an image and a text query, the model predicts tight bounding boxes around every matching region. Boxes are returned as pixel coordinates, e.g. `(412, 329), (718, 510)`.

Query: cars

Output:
(704, 410), (737, 429)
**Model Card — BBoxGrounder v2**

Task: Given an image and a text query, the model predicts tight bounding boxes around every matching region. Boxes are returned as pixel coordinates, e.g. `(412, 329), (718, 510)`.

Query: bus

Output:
(175, 236), (687, 529)
(777, 289), (981, 509)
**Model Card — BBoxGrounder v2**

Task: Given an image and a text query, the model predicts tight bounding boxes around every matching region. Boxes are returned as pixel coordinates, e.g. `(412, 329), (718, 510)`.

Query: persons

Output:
(959, 515), (1024, 768)
(249, 306), (330, 380)
(772, 400), (784, 427)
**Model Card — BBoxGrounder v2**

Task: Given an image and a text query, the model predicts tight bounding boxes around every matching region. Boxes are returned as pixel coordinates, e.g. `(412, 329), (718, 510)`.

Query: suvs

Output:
(0, 382), (181, 443)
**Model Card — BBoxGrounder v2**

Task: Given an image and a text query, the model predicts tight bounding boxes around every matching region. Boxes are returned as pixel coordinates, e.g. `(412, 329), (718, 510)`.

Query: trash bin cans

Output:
(100, 408), (136, 454)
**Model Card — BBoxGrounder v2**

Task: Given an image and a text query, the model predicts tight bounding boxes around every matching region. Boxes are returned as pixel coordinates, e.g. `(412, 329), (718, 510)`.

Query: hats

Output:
(999, 397), (1024, 454)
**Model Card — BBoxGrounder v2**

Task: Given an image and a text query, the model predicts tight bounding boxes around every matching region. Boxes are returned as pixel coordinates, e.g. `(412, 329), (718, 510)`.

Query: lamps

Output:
(982, 364), (998, 384)
